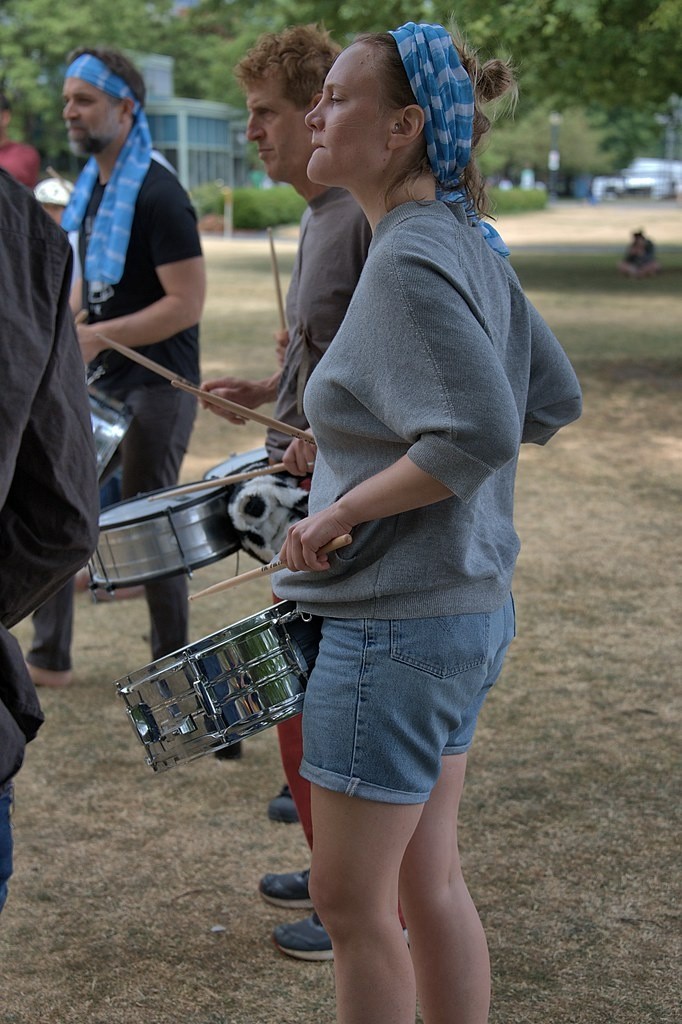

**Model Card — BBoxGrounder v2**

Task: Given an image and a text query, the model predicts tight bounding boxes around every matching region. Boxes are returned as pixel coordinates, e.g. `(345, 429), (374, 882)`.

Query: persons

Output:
(300, 20), (581, 1024)
(618, 230), (661, 282)
(202, 18), (373, 957)
(0, 73), (102, 918)
(24, 45), (206, 688)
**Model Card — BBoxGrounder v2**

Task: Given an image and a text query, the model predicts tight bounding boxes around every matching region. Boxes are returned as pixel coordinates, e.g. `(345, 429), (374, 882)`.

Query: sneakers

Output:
(261, 870), (314, 908)
(274, 913), (409, 960)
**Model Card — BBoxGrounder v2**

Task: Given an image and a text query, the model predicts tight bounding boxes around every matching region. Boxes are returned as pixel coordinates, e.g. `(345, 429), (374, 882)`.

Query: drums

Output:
(84, 480), (241, 588)
(204, 447), (268, 482)
(85, 384), (134, 482)
(115, 598), (323, 776)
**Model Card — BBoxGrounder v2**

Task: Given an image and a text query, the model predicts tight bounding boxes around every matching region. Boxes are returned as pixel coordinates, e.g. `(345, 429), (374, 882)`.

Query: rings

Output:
(306, 460), (316, 467)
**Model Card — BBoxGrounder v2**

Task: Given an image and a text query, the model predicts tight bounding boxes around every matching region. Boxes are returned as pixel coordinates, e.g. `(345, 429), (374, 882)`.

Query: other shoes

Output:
(268, 785), (299, 822)
